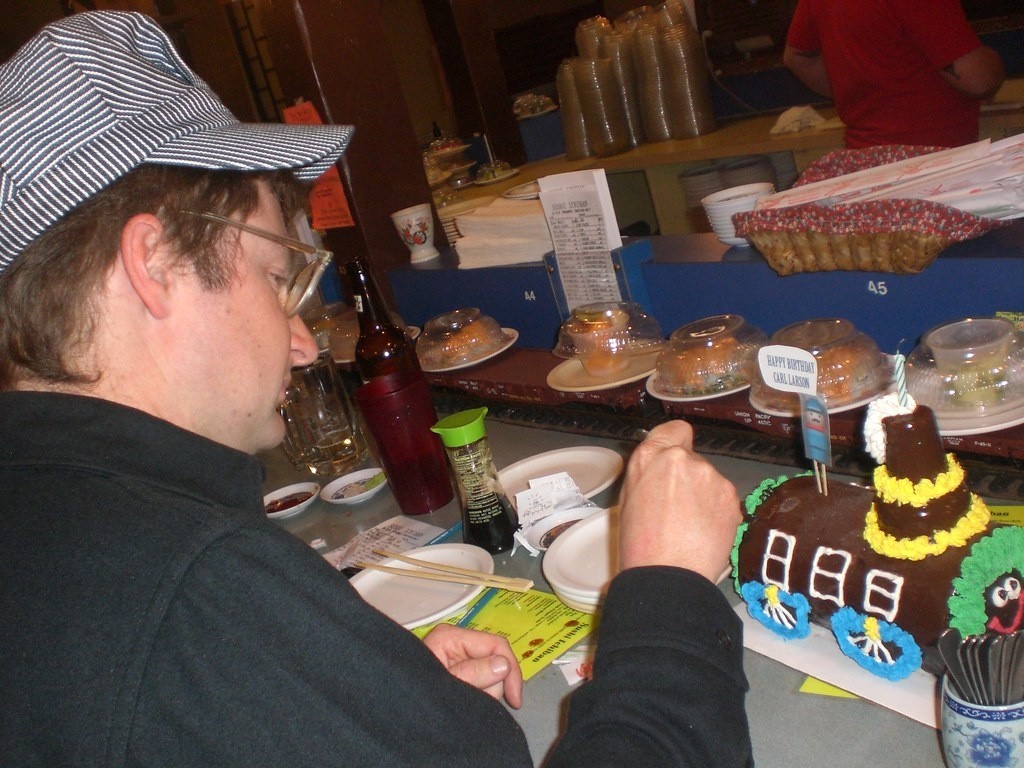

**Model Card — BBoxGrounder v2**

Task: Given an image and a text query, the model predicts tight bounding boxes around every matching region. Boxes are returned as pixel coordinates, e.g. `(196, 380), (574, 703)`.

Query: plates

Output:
(410, 328), (1024, 435)
(679, 152), (800, 250)
(526, 506), (737, 615)
(258, 482), (321, 519)
(495, 446), (624, 518)
(320, 468), (388, 505)
(421, 92), (557, 249)
(332, 326), (421, 365)
(347, 543), (495, 630)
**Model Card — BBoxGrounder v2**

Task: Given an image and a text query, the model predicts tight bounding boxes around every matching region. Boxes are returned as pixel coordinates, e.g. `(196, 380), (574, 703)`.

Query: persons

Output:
(783, 0), (1004, 149)
(0, 11), (755, 768)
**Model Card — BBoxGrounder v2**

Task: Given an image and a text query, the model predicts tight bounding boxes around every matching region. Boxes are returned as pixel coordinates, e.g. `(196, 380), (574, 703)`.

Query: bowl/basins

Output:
(295, 302), (407, 359)
(416, 306), (1024, 422)
(553, 0), (716, 161)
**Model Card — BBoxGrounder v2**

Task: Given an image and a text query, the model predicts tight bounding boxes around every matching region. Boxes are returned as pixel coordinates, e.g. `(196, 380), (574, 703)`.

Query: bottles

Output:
(345, 254), (436, 420)
(429, 405), (519, 553)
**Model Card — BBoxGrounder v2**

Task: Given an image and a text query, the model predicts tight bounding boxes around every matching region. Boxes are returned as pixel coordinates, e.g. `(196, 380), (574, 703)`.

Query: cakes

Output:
(730, 394), (1024, 682)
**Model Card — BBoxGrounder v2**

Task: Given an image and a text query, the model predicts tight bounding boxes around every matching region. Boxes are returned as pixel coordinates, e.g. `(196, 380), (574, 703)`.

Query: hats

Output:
(0, 8), (355, 278)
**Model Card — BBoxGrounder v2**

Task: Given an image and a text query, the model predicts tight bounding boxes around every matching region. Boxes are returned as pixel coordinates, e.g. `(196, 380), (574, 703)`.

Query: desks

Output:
(258, 411), (1024, 768)
(457, 78), (1024, 237)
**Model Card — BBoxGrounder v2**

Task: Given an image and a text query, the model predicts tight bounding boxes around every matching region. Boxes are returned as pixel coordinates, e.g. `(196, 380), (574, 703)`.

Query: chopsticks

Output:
(353, 549), (534, 594)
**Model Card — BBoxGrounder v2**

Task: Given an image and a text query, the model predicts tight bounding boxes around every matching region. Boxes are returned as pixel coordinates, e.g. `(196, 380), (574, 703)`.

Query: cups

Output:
(270, 353), (369, 470)
(390, 202), (440, 263)
(353, 359), (453, 515)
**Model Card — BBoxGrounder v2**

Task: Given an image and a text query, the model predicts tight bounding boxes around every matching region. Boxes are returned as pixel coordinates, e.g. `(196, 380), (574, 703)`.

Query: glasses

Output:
(150, 204), (334, 318)
